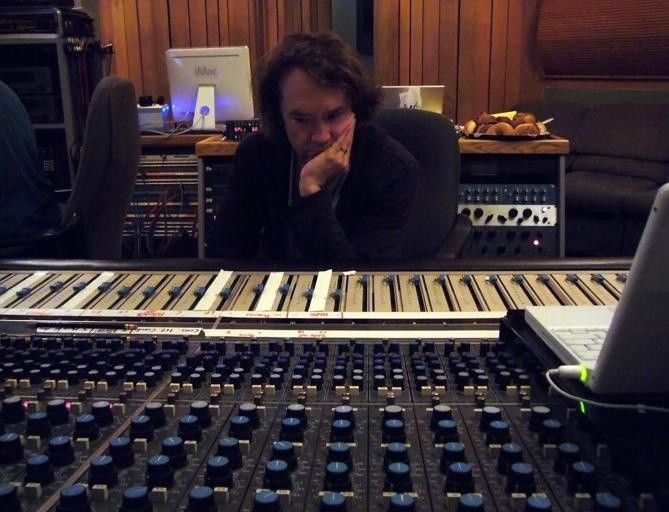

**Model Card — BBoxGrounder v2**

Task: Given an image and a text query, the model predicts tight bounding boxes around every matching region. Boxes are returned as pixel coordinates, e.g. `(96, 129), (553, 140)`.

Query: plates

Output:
(459, 130), (551, 140)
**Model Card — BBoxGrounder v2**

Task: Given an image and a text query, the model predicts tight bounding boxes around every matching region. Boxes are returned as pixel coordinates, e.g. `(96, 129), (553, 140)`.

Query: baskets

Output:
(225, 118), (263, 141)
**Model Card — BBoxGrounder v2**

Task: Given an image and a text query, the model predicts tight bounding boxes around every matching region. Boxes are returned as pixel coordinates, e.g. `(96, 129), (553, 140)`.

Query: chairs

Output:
(566, 103), (668, 258)
(1, 76), (141, 259)
(469, 99), (588, 182)
(372, 108), (473, 261)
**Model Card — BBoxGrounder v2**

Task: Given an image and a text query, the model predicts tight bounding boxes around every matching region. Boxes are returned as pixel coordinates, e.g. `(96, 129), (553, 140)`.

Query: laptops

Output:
(524, 182), (669, 396)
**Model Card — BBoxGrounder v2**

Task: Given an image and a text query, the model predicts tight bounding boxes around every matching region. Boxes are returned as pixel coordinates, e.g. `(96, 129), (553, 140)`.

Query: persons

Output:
(1, 80), (64, 258)
(225, 33), (429, 269)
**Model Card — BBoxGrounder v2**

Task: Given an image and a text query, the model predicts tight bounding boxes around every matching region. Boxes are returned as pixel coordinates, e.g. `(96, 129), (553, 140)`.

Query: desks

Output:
(194, 131), (569, 262)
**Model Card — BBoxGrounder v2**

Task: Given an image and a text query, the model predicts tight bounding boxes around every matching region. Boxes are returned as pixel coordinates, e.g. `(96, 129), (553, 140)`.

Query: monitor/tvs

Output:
(165, 46), (255, 132)
(380, 84), (445, 116)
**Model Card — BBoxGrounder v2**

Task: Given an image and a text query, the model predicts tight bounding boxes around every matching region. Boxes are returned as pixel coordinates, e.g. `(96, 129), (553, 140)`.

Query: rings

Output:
(340, 147), (351, 154)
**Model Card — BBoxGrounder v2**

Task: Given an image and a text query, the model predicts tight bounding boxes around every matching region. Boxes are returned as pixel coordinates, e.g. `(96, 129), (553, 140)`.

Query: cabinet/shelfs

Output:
(0, 7), (105, 192)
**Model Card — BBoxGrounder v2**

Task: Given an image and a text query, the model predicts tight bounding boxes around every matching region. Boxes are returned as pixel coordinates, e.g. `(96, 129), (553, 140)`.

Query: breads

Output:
(463, 112), (540, 135)
